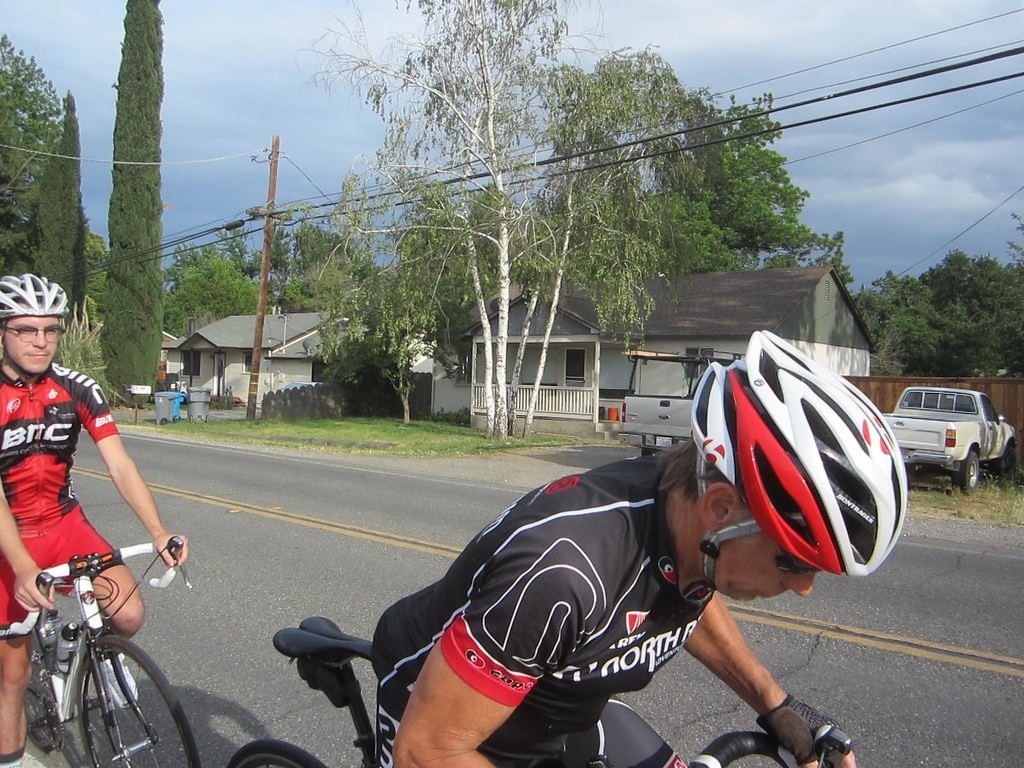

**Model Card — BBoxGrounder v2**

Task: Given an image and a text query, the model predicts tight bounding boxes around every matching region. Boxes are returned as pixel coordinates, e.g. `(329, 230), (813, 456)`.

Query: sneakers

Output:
(100, 652), (138, 708)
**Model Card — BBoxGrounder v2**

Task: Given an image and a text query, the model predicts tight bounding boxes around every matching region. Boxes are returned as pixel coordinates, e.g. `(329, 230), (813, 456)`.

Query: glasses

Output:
(773, 546), (822, 574)
(0, 326), (66, 343)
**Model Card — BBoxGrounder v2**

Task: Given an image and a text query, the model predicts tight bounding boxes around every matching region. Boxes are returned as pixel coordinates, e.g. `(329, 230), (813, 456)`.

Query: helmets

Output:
(0, 273), (70, 319)
(690, 330), (907, 576)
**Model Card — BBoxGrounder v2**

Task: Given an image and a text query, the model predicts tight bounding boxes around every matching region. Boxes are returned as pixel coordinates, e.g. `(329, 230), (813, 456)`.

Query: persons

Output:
(370, 329), (907, 767)
(0, 274), (189, 768)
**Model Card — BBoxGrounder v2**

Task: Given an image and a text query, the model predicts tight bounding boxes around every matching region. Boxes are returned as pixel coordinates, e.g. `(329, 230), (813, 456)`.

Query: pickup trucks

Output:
(879, 384), (1019, 496)
(618, 348), (748, 458)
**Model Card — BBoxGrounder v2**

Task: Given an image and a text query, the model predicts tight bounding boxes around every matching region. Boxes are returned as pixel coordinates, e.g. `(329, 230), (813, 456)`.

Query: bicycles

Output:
(223, 613), (853, 768)
(0, 535), (204, 768)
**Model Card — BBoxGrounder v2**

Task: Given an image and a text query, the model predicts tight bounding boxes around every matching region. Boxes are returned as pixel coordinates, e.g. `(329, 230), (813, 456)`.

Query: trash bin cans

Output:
(608, 407), (618, 421)
(598, 407), (605, 420)
(186, 387), (211, 422)
(154, 393), (177, 425)
(159, 392), (186, 422)
